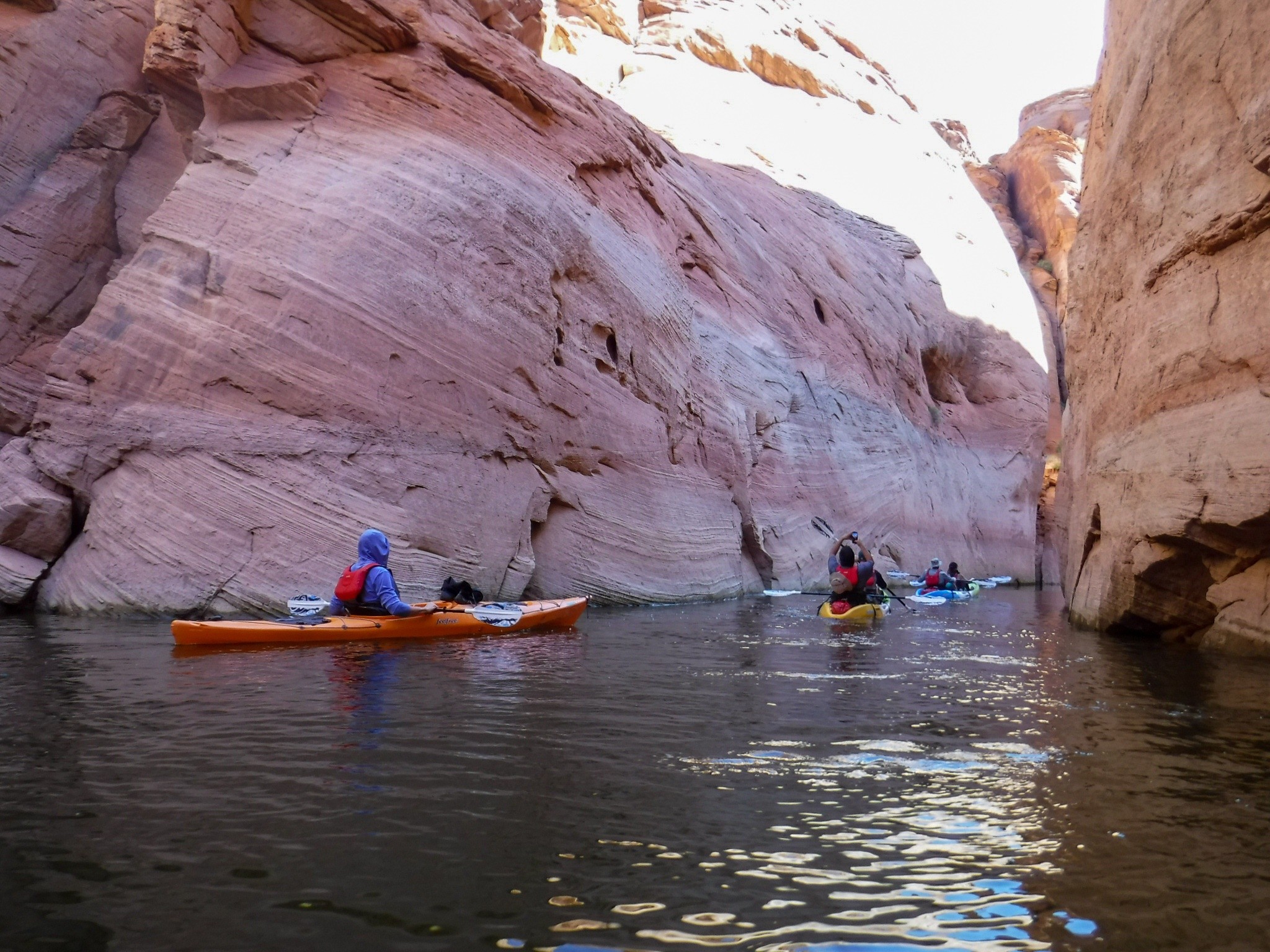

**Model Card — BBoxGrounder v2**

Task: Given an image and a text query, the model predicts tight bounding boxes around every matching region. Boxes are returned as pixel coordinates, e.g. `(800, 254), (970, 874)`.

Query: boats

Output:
(915, 583), (980, 600)
(818, 587), (891, 622)
(170, 594), (595, 646)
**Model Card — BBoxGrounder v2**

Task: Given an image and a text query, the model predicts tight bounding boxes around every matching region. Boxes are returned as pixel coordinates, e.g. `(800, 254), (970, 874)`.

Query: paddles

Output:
(810, 515), (917, 611)
(762, 589), (947, 607)
(287, 593), (524, 628)
(909, 575), (1012, 587)
(886, 570), (996, 589)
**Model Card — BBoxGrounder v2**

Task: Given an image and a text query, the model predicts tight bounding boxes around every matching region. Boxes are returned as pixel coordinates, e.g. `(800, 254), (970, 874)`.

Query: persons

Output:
(917, 558), (970, 592)
(828, 531), (888, 607)
(329, 529), (438, 617)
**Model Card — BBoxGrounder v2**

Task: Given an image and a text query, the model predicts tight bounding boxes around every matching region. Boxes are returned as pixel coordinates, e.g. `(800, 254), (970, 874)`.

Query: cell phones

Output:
(851, 532), (859, 544)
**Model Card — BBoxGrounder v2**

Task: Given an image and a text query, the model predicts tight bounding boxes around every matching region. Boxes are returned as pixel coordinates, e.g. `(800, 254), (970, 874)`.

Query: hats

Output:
(829, 572), (853, 594)
(929, 558), (942, 567)
